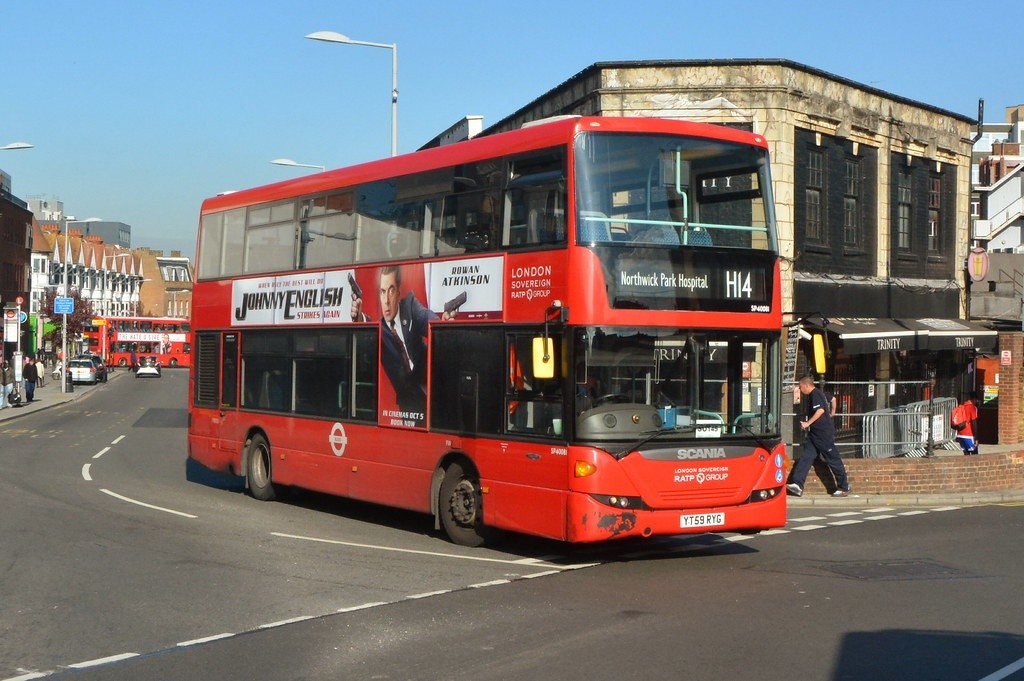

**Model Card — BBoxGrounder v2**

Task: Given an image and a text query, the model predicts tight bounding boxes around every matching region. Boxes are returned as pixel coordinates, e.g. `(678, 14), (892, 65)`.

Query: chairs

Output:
(224, 209), (714, 276)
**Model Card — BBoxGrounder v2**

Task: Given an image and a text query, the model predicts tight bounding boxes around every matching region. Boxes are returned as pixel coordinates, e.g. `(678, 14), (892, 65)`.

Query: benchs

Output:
(258, 369), (376, 421)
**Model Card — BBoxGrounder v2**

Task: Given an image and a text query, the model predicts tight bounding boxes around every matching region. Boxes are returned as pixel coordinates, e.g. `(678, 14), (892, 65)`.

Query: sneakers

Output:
(833, 486), (852, 497)
(787, 483), (803, 497)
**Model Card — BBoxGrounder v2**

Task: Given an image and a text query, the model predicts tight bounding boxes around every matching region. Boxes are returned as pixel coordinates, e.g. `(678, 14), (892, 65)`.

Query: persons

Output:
(128, 349), (136, 372)
(161, 334), (172, 354)
(0, 353), (44, 409)
(786, 375), (852, 497)
(351, 265), (457, 428)
(956, 391), (981, 455)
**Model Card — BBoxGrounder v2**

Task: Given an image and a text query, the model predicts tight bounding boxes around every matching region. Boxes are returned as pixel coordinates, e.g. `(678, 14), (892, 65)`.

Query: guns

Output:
(347, 273), (362, 322)
(444, 291), (467, 315)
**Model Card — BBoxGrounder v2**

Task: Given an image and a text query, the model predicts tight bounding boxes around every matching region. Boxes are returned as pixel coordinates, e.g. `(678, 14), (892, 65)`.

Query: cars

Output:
(134, 356), (162, 379)
(66, 353), (108, 386)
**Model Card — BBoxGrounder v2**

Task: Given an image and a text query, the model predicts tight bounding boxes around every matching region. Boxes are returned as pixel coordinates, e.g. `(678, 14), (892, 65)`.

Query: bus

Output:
(182, 115), (832, 547)
(74, 313), (191, 369)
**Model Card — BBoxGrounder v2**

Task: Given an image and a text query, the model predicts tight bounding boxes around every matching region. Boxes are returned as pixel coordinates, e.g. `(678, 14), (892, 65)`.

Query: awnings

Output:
(806, 318), (998, 355)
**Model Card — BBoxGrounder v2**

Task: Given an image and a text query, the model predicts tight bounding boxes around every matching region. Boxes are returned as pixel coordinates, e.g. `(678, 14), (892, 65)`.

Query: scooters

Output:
(52, 361), (63, 380)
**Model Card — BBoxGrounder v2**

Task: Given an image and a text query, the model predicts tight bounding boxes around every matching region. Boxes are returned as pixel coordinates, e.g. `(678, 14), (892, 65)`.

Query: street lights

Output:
(303, 31), (398, 158)
(133, 279), (152, 317)
(59, 217), (106, 393)
(102, 253), (130, 361)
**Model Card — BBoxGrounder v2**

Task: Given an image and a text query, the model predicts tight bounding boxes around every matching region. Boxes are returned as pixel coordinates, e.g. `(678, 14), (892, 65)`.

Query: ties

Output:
(390, 320), (412, 376)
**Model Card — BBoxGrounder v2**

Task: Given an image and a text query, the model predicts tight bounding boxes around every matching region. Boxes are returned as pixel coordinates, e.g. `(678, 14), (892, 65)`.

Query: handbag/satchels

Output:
(8, 387), (21, 404)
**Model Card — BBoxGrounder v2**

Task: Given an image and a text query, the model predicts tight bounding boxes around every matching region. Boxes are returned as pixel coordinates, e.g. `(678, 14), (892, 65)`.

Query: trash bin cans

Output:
(66, 372), (72, 393)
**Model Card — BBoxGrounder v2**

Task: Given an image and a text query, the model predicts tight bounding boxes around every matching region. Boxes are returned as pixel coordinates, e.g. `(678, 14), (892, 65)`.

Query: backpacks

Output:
(951, 402), (973, 430)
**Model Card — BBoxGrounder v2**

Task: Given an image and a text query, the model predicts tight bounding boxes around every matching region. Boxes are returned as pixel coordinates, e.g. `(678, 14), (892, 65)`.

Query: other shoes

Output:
(6, 403), (12, 408)
(27, 401), (32, 403)
(0, 407), (3, 409)
